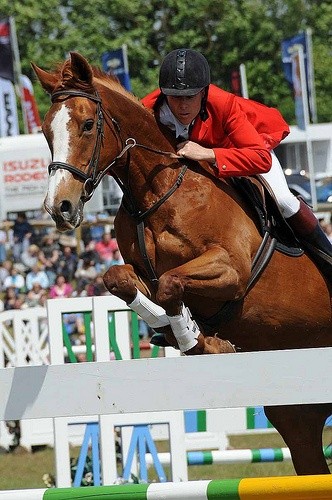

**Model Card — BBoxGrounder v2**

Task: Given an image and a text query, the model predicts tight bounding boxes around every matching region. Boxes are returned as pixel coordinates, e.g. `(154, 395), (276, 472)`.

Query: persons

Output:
(0, 209), (128, 309)
(134, 47), (332, 347)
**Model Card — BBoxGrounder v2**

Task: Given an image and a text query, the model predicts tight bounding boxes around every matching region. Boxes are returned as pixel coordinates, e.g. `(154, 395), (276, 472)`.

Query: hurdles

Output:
(0, 296), (332, 499)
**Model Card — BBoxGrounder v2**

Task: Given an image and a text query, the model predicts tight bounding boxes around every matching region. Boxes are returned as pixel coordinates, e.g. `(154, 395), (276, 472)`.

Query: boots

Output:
(149, 332), (172, 347)
(301, 225), (332, 305)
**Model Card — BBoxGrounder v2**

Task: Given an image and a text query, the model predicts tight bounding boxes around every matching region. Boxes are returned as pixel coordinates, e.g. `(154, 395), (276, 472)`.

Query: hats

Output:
(159, 49), (210, 95)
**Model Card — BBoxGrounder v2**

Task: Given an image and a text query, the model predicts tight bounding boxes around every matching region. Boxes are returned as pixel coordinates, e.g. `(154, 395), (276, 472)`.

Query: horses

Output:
(30, 51), (332, 476)
